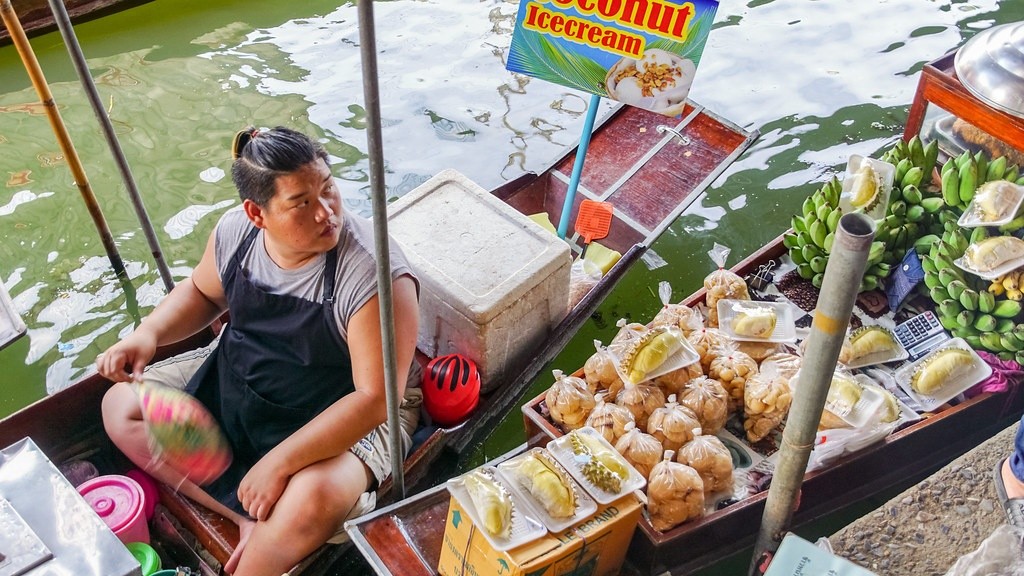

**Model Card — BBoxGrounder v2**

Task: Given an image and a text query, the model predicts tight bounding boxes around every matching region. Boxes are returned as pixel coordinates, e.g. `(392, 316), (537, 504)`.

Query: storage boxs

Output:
(436, 492), (643, 576)
(366, 168), (575, 397)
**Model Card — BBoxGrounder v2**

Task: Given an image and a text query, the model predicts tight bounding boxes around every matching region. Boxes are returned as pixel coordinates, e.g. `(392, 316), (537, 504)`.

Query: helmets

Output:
(422, 352), (481, 426)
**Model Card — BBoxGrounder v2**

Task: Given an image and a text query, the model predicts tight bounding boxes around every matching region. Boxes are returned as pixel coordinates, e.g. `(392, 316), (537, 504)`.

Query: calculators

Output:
(892, 310), (951, 362)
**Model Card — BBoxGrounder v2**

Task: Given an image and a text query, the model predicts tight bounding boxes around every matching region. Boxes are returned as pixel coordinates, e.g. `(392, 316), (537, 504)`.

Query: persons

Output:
(96, 126), (425, 576)
(992, 413), (1024, 551)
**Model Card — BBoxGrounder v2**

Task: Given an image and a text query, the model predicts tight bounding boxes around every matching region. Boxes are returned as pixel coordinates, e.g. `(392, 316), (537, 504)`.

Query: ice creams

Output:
(602, 49), (695, 117)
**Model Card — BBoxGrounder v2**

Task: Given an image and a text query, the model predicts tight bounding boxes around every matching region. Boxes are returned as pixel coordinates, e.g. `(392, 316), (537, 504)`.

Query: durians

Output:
(464, 467), (517, 540)
(971, 178), (1019, 221)
(514, 449), (580, 518)
(873, 393), (904, 422)
(909, 347), (975, 394)
(570, 429), (630, 494)
(850, 166), (884, 212)
(730, 307), (778, 339)
(962, 235), (1024, 272)
(618, 325), (685, 383)
(827, 376), (862, 405)
(843, 323), (898, 361)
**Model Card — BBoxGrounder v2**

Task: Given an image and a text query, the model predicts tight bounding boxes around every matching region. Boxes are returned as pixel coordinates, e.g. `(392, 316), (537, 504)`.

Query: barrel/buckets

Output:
(75, 475), (151, 547)
(118, 540), (162, 575)
(150, 569), (191, 576)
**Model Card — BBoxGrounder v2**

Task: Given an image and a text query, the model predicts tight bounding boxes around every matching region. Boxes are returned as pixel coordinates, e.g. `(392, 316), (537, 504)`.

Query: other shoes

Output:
(993, 456), (1024, 551)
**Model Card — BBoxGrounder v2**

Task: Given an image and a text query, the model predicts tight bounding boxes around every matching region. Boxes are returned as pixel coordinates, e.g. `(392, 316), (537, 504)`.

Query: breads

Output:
(544, 272), (791, 532)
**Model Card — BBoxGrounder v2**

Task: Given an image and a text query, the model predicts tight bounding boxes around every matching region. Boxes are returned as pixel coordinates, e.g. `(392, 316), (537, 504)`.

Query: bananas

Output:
(783, 134), (1024, 368)
